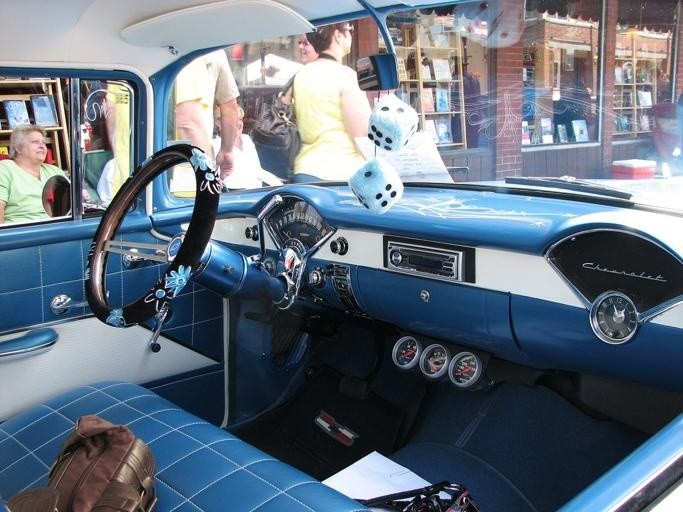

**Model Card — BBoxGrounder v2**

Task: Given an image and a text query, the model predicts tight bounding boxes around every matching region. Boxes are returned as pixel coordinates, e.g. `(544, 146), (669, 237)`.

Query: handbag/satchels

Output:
(249, 73), (301, 180)
(7, 414), (158, 512)
(356, 480), (480, 511)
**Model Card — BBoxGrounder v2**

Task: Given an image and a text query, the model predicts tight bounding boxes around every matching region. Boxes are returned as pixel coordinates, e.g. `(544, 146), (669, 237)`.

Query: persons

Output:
(657, 67), (670, 102)
(105, 49), (262, 198)
(278, 21), (372, 183)
(43, 177), (57, 217)
(0, 125), (90, 224)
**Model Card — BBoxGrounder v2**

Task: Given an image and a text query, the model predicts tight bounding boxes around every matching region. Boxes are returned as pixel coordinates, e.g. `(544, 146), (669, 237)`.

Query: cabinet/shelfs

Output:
(378, 19), (468, 151)
(614, 58), (658, 138)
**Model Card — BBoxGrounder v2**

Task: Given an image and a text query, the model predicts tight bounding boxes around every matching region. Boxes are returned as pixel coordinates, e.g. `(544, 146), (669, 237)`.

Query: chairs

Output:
(0, 382), (367, 512)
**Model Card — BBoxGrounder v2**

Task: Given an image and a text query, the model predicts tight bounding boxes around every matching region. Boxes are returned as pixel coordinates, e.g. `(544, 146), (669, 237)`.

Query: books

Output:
(395, 57), (453, 145)
(3, 100), (30, 130)
(30, 95), (59, 126)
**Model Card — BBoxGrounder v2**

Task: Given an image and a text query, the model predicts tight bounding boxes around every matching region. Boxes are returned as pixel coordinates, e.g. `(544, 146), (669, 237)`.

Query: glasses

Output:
(298, 39), (309, 46)
(336, 24), (355, 31)
